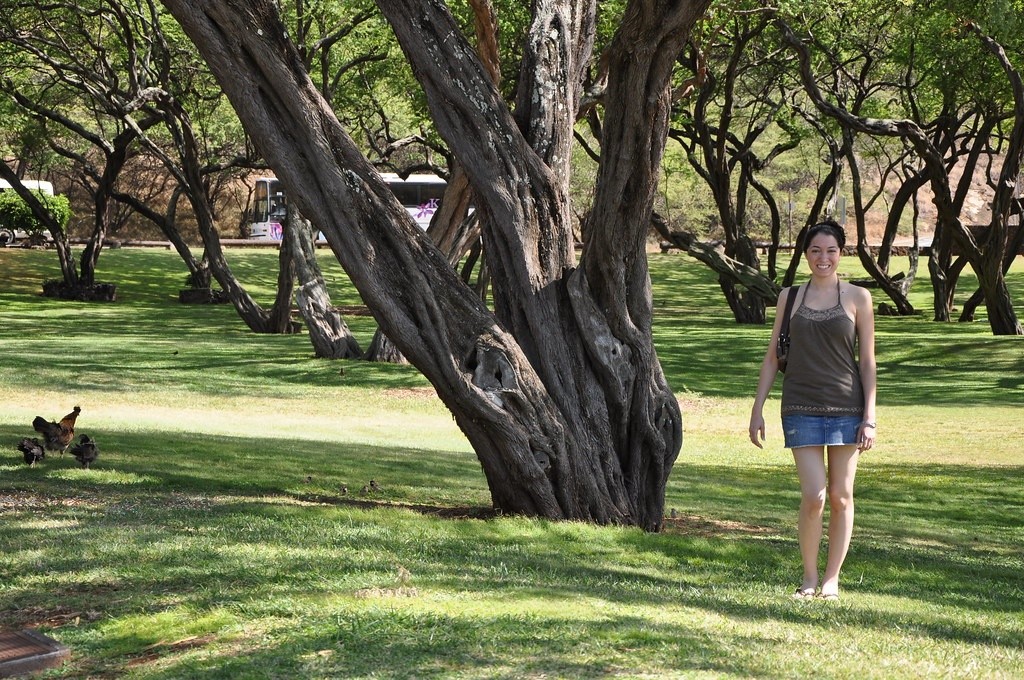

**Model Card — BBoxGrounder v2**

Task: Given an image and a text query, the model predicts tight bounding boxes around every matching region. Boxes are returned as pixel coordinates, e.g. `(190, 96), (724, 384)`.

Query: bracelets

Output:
(861, 420), (877, 429)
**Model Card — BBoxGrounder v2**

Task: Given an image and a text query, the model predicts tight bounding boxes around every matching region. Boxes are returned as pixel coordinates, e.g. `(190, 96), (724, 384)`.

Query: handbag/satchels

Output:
(776, 285), (800, 372)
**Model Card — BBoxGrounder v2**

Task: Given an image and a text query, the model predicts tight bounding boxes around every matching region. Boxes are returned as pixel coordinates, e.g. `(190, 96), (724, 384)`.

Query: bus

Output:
(0, 178), (57, 244)
(247, 171), (478, 252)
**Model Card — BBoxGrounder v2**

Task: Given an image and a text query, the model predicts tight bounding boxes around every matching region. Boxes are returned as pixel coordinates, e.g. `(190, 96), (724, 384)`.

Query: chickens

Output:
(33, 406), (81, 459)
(17, 437), (45, 469)
(69, 434), (98, 469)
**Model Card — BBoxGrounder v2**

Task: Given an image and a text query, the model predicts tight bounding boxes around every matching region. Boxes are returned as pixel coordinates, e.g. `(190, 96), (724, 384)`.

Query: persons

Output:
(749, 220), (878, 604)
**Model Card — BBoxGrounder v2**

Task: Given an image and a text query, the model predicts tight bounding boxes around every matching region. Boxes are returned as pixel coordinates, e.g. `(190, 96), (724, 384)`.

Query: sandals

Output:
(795, 588), (814, 600)
(816, 592), (839, 601)
